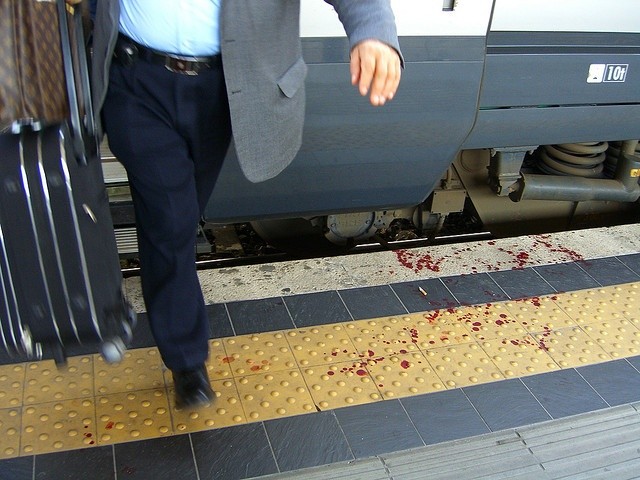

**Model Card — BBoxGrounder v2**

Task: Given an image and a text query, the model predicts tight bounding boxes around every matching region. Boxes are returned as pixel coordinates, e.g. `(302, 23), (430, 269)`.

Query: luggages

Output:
(0, 1), (139, 371)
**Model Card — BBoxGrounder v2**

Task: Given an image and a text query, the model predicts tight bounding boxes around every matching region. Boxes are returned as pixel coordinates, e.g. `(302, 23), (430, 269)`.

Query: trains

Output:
(201, 0), (639, 247)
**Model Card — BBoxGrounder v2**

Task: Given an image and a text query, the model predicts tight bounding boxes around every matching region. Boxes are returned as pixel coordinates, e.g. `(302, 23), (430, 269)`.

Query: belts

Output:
(112, 35), (228, 75)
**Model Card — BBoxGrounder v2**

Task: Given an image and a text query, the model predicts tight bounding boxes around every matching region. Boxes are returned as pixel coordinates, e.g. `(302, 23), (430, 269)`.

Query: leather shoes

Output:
(170, 364), (218, 411)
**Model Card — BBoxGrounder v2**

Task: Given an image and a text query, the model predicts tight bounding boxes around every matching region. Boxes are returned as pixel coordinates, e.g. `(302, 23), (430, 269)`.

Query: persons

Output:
(89, 0), (405, 412)
